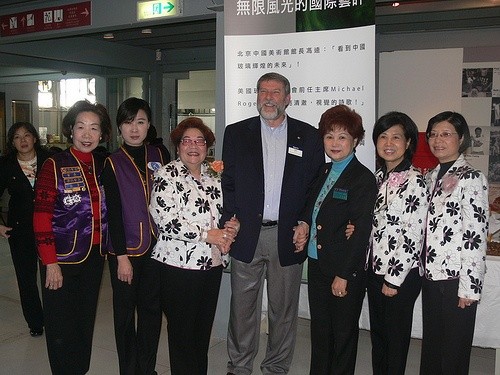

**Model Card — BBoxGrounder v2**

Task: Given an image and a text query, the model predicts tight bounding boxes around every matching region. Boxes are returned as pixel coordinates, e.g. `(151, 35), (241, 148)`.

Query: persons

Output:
(470, 127), (486, 154)
(344, 111), (432, 374)
(293, 103), (376, 375)
(97, 98), (172, 373)
(219, 73), (328, 375)
(150, 117), (241, 375)
(34, 100), (122, 375)
(417, 110), (489, 375)
(0, 121), (59, 336)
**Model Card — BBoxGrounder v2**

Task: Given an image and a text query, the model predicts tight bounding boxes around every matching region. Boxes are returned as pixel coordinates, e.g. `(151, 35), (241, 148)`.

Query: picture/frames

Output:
(11, 100), (33, 125)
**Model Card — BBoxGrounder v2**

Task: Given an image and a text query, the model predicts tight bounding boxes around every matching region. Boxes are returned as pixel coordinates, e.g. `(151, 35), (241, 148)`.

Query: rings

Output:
(338, 291), (343, 294)
(223, 233), (227, 239)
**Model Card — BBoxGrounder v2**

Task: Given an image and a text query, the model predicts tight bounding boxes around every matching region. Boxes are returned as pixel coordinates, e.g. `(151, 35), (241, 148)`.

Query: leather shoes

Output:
(30, 324), (43, 336)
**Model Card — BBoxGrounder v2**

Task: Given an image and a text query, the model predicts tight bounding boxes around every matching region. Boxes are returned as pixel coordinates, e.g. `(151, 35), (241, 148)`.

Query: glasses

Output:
(426, 130), (458, 139)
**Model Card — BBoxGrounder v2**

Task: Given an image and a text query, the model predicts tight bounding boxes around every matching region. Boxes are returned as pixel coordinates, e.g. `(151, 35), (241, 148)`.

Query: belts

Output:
(260, 221), (277, 226)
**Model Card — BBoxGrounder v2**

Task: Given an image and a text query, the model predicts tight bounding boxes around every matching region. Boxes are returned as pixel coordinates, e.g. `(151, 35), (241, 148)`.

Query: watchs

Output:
(201, 229), (208, 244)
